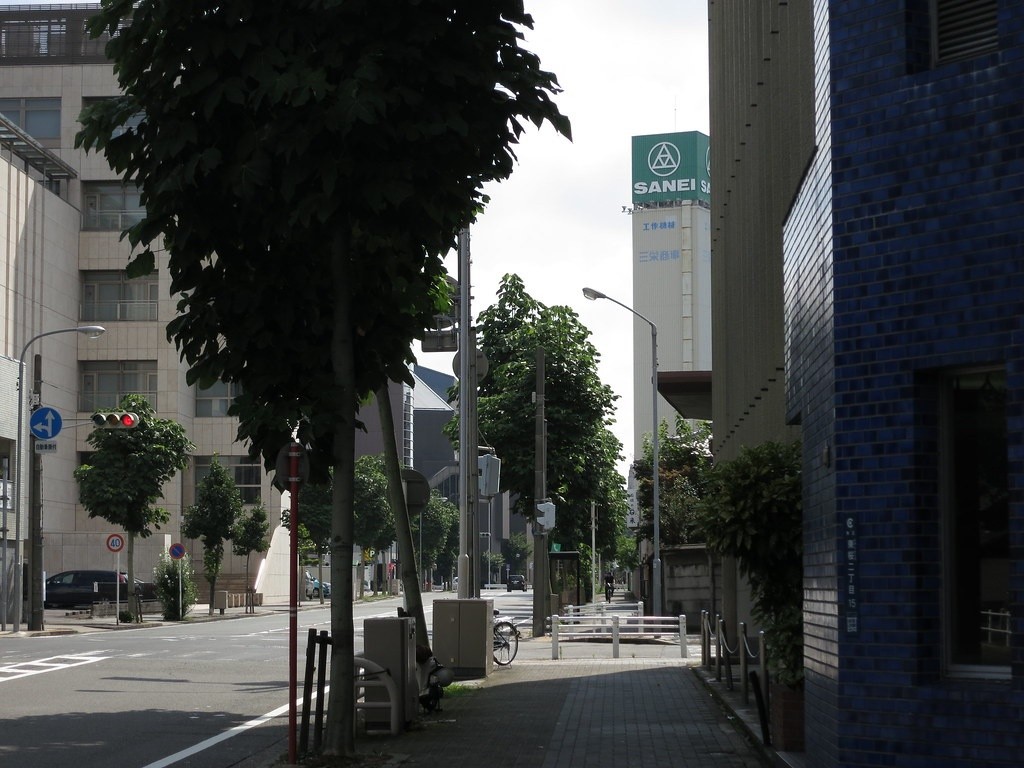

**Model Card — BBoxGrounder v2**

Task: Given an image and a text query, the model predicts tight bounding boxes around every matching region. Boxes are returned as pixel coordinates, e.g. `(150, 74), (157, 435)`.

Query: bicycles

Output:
(604, 583), (614, 604)
(492, 609), (522, 669)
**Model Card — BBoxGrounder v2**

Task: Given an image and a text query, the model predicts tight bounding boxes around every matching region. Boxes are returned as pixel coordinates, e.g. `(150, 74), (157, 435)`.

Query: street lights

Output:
(582, 288), (663, 641)
(28, 325), (106, 632)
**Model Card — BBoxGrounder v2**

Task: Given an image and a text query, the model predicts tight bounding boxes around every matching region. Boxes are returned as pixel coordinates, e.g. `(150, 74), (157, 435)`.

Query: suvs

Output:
(43, 569), (154, 608)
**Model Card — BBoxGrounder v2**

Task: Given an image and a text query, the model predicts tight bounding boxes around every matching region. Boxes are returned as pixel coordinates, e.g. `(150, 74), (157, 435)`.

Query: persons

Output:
(603, 572), (614, 601)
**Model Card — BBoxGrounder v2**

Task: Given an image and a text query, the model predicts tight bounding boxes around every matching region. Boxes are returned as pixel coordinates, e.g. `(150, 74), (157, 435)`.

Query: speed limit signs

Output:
(107, 534), (125, 552)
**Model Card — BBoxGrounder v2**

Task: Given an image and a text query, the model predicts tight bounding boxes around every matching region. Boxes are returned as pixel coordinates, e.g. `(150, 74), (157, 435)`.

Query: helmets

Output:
(433, 666), (455, 688)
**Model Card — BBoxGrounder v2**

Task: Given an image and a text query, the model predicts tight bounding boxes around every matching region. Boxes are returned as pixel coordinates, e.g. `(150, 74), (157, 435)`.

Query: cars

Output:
(305, 571), (331, 599)
(505, 574), (527, 592)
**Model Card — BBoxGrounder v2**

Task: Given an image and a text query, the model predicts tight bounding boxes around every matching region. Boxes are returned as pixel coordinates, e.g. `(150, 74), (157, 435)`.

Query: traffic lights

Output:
(536, 502), (556, 530)
(92, 412), (140, 429)
(479, 454), (501, 497)
